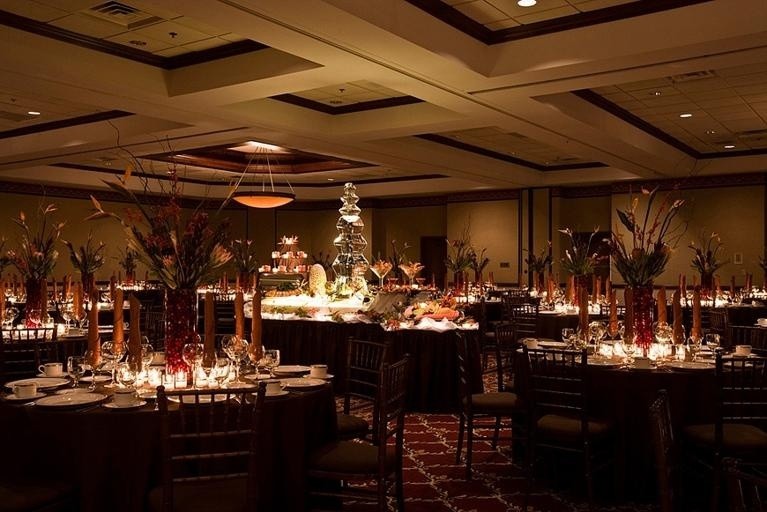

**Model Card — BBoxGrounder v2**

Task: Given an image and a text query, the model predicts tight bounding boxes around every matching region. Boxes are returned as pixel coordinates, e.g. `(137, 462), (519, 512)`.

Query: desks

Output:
(508, 332), (765, 427)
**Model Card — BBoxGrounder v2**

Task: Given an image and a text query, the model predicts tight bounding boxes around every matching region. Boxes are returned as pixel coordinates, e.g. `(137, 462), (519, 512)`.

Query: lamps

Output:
(225, 142), (297, 215)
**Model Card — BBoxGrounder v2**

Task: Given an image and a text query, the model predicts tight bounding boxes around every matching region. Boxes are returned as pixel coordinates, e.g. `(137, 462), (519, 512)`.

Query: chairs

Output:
(149, 382), (271, 512)
(517, 340), (612, 511)
(687, 350), (764, 464)
(289, 350), (415, 510)
(323, 334), (393, 451)
(489, 323), (525, 450)
(451, 327), (520, 483)
(646, 385), (713, 509)
(721, 455), (762, 511)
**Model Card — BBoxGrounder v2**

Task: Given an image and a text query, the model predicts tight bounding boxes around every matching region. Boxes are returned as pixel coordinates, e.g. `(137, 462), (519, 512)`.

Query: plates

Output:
(538, 340), (566, 348)
(516, 348), (548, 356)
(102, 400), (148, 408)
(282, 377), (331, 390)
(273, 364), (313, 376)
(303, 373), (336, 380)
(34, 392), (109, 408)
(163, 393), (238, 404)
(55, 389), (95, 394)
(252, 392), (291, 396)
(4, 377), (72, 388)
(5, 392), (48, 401)
(35, 371), (68, 377)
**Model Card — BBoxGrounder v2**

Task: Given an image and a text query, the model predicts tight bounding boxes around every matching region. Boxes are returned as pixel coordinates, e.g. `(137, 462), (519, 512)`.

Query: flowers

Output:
(0, 115), (258, 297)
(381, 148), (728, 287)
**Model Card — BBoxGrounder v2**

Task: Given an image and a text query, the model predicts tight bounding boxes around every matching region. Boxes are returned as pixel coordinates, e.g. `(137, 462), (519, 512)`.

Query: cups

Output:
(4, 288), (113, 308)
(524, 338), (538, 350)
(462, 280), (621, 311)
(261, 379), (287, 394)
(112, 387), (138, 407)
(116, 279), (220, 292)
(38, 362), (64, 376)
(665, 285), (765, 304)
(13, 382), (37, 398)
(310, 364), (330, 378)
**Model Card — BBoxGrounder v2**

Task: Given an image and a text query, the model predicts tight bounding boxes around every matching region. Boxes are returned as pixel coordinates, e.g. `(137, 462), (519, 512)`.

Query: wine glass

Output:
(1, 305), (87, 337)
(402, 268), (421, 288)
(561, 318), (755, 370)
(65, 335), (282, 388)
(369, 266), (391, 288)
